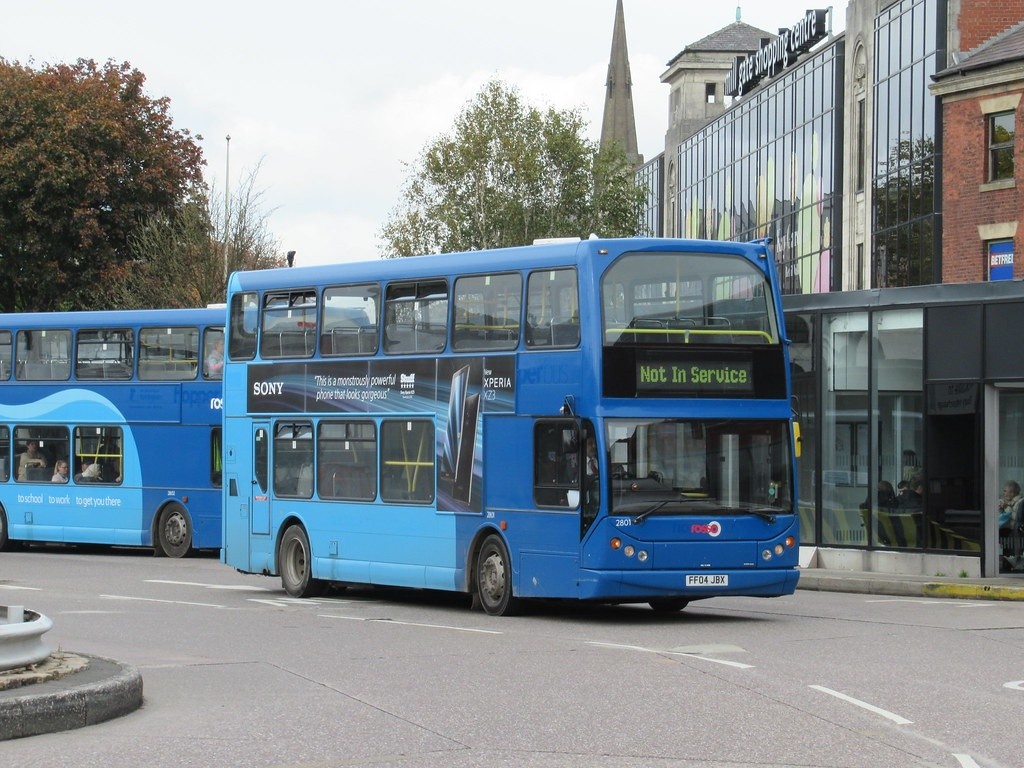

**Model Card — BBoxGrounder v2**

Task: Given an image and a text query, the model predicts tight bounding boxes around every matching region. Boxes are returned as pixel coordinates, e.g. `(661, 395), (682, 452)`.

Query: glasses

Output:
(60, 466), (68, 469)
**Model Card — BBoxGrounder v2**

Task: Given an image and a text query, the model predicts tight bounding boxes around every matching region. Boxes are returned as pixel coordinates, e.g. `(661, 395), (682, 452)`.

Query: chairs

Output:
(6, 456), (24, 481)
(332, 470), (367, 499)
(374, 475), (411, 500)
(542, 461), (572, 505)
(1, 357), (195, 384)
(259, 316), (734, 358)
(27, 468), (56, 483)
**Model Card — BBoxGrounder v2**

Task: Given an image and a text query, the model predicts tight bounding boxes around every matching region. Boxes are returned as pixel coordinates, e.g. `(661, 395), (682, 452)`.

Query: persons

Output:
(861, 475), (1023, 567)
(18, 438), (121, 484)
(207, 338), (223, 377)
(566, 438), (598, 485)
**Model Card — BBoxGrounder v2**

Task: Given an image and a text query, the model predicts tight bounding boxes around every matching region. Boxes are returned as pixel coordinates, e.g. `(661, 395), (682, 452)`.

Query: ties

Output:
(589, 460), (598, 474)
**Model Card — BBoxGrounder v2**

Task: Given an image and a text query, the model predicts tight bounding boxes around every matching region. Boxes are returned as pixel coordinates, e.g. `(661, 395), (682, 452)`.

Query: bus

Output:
(221, 235), (805, 619)
(1, 301), (373, 561)
